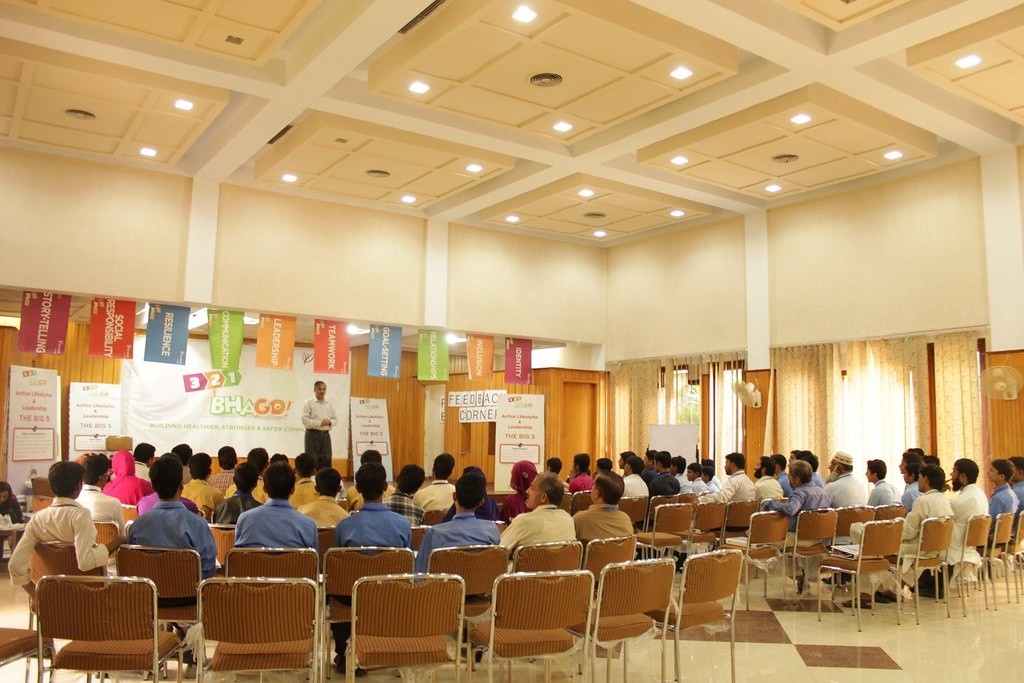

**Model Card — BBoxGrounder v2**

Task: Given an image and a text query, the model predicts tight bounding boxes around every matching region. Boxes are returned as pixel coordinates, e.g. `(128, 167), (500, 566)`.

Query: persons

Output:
(841, 465), (953, 608)
(759, 460), (831, 592)
(571, 470), (634, 538)
(502, 471), (577, 573)
(6, 460), (127, 614)
(76, 444), (946, 525)
(1, 481), (23, 525)
(1008, 456), (1024, 543)
(128, 458), (217, 679)
(413, 472), (501, 601)
(302, 381), (334, 465)
(976, 459), (1018, 549)
(911, 459), (989, 599)
(327, 461), (412, 678)
(234, 461), (319, 550)
(821, 452), (866, 586)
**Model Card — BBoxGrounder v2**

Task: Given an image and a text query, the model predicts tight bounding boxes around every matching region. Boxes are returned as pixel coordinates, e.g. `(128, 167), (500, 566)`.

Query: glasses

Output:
(99, 470), (113, 478)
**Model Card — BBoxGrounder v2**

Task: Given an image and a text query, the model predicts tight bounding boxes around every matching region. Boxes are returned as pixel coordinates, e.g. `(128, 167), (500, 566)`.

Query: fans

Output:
(730, 380), (762, 409)
(976, 365), (1024, 403)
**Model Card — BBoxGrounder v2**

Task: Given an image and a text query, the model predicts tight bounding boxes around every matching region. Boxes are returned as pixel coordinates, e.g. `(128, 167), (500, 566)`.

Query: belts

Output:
(309, 428), (329, 434)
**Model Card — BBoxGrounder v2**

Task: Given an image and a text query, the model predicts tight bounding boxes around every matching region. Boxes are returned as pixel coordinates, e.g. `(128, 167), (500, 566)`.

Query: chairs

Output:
(0, 435), (1024, 682)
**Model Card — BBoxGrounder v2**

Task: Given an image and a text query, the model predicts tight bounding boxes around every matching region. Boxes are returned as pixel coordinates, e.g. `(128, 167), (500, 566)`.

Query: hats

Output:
(834, 450), (854, 465)
